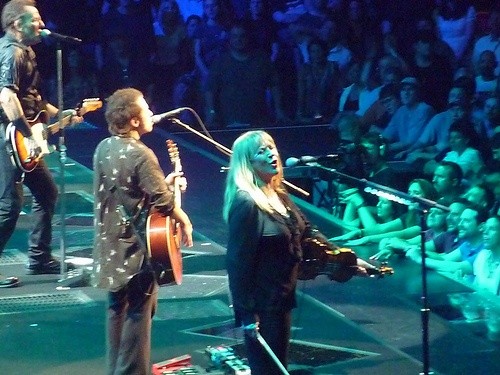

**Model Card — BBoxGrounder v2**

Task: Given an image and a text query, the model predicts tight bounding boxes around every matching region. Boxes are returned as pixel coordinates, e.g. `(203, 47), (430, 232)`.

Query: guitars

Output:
(6, 98), (102, 173)
(146, 140), (182, 287)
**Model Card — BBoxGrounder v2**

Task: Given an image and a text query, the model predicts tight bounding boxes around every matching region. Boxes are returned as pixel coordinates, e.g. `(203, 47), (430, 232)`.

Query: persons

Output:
(223, 131), (378, 375)
(0, 0), (84, 287)
(38, 0), (500, 298)
(92, 88), (193, 375)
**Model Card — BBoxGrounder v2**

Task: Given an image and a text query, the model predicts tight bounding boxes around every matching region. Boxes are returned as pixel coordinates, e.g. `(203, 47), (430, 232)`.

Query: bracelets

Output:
(55, 111), (60, 117)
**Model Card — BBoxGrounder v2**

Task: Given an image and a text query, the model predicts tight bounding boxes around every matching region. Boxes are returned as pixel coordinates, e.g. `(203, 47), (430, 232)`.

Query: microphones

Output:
(286, 155), (337, 168)
(154, 108), (184, 123)
(40, 29), (81, 43)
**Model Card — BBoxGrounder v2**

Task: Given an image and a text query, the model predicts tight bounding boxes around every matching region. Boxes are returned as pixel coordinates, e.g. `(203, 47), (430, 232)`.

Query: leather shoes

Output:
(26, 259), (74, 275)
(0, 275), (20, 287)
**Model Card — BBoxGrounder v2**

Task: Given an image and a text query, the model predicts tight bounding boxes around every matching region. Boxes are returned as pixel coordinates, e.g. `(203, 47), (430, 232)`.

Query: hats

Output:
(400, 77), (420, 88)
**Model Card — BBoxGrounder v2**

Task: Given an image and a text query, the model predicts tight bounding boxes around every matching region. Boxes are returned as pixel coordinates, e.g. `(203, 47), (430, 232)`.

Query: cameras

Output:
(340, 136), (364, 155)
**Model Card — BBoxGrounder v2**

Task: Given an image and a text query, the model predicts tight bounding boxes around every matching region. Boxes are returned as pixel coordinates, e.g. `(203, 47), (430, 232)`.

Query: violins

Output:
(297, 238), (394, 283)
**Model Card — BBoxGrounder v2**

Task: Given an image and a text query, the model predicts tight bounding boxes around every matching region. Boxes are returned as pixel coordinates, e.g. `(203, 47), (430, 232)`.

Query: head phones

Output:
(379, 134), (386, 156)
